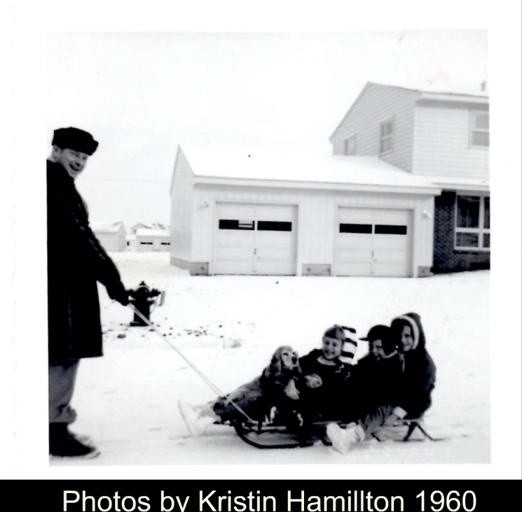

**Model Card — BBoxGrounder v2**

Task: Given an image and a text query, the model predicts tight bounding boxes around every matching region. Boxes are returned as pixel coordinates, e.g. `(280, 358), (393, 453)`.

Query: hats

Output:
(358, 325), (398, 355)
(52, 127), (98, 155)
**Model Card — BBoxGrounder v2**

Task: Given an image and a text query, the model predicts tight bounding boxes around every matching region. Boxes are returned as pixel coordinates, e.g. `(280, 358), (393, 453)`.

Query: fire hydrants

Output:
(127, 280), (161, 326)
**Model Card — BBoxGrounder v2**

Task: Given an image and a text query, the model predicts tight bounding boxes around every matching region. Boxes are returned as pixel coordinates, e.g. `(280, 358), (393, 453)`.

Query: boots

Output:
(49, 423), (93, 456)
(327, 421), (366, 455)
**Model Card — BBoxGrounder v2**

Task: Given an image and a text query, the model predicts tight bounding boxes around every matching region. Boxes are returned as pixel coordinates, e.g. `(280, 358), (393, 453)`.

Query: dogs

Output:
(212, 345), (302, 415)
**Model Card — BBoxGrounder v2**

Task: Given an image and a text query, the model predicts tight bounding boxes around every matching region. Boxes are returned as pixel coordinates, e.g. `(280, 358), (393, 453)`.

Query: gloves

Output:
(106, 281), (129, 306)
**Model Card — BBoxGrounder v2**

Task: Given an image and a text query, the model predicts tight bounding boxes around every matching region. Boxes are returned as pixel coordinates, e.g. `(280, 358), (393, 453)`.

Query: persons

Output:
(267, 311), (437, 455)
(46, 126), (131, 460)
